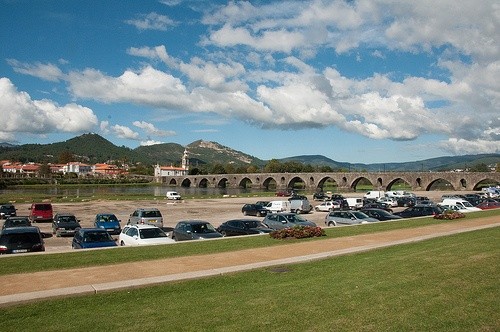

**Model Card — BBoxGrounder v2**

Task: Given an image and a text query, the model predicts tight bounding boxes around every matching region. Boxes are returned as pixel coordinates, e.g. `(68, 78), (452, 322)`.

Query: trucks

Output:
(287, 194), (312, 214)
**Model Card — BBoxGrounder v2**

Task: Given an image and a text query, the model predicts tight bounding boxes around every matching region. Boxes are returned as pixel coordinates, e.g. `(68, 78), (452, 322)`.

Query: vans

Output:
(27, 202), (54, 223)
(262, 201), (291, 213)
(166, 191), (181, 200)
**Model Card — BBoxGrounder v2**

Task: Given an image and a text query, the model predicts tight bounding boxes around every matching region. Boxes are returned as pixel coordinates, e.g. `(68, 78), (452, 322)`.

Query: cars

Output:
(0, 204), (18, 220)
(172, 220), (225, 242)
(2, 217), (33, 230)
(216, 218), (274, 237)
(313, 186), (500, 227)
(274, 190), (291, 197)
(0, 226), (48, 254)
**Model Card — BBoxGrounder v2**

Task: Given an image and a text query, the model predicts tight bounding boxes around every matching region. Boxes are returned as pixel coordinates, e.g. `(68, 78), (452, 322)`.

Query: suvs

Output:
(242, 204), (272, 217)
(262, 212), (317, 231)
(127, 207), (163, 231)
(71, 227), (118, 249)
(324, 210), (380, 227)
(118, 223), (175, 247)
(256, 201), (270, 207)
(52, 213), (82, 237)
(94, 213), (122, 235)
